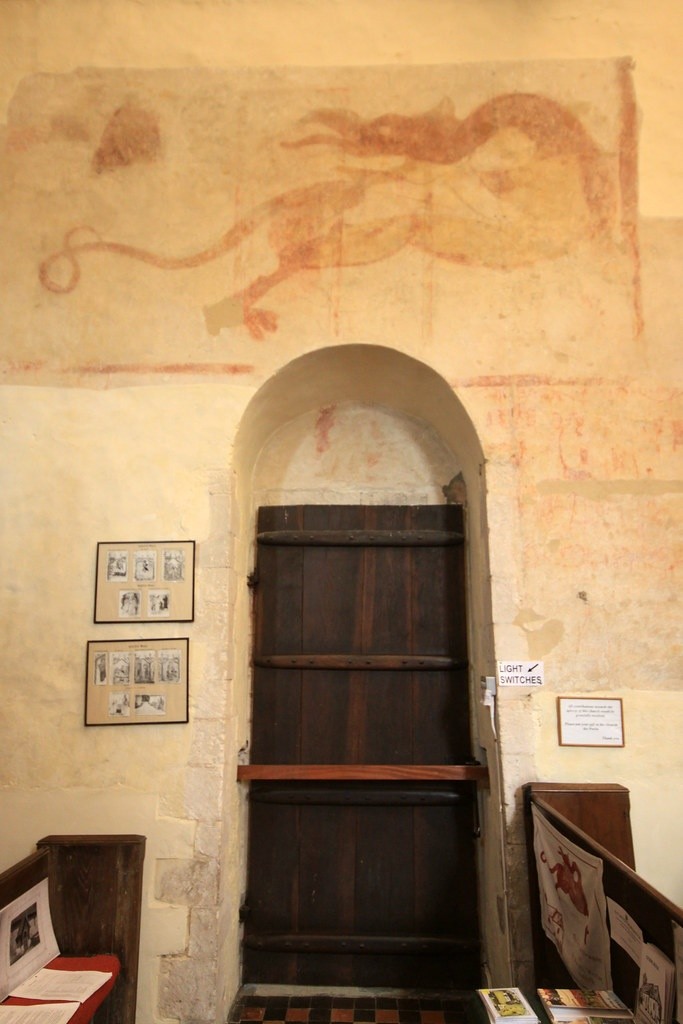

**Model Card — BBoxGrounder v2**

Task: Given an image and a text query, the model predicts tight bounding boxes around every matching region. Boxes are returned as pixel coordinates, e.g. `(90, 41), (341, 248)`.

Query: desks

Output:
(0, 953), (121, 1024)
(460, 992), (550, 1024)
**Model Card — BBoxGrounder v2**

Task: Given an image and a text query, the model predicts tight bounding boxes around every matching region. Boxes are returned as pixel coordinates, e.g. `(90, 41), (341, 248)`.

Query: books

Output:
(478, 943), (675, 1024)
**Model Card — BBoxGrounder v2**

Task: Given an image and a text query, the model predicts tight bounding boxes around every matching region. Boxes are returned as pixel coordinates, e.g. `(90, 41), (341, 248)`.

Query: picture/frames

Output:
(84, 638), (190, 726)
(93, 540), (194, 624)
